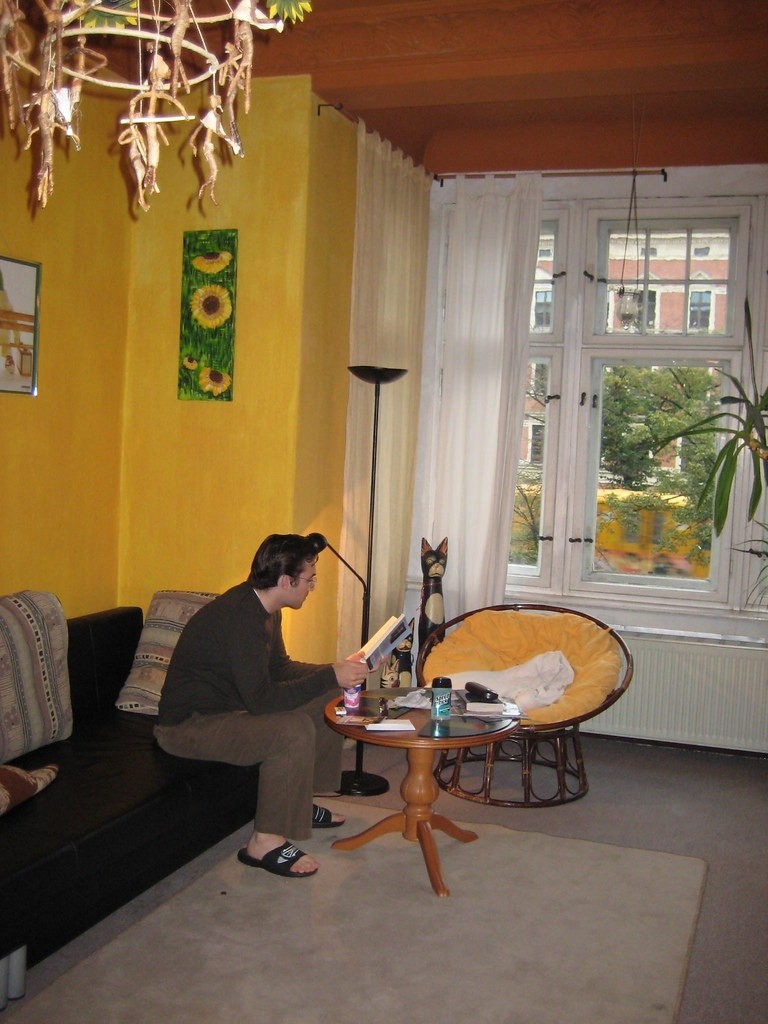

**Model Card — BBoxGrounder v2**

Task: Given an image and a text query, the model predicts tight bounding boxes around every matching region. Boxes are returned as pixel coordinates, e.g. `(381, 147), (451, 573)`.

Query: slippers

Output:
(312, 804), (346, 828)
(237, 840), (318, 877)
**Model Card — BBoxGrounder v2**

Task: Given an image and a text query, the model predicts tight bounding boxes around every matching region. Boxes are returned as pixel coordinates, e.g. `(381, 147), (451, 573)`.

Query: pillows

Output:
(0, 590), (73, 765)
(115, 589), (221, 715)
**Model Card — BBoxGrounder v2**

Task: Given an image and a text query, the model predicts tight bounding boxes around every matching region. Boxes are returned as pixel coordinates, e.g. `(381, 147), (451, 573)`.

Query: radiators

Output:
(578, 634), (768, 754)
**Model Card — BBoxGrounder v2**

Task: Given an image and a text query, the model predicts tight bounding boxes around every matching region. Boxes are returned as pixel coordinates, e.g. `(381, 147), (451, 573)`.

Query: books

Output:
(357, 614), (412, 670)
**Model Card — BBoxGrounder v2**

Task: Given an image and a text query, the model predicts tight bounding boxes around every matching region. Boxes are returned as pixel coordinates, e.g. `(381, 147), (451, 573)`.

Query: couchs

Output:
(0, 607), (263, 1010)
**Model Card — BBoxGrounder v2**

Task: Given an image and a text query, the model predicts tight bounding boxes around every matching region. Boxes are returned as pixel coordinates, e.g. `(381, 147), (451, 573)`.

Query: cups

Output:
(344, 684), (361, 708)
(432, 677), (452, 719)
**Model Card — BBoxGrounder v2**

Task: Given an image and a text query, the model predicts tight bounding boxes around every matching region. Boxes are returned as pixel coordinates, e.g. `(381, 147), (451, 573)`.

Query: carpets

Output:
(0, 800), (708, 1024)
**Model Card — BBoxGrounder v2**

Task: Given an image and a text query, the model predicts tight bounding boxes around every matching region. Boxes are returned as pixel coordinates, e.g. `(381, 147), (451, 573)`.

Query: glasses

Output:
(290, 574), (319, 589)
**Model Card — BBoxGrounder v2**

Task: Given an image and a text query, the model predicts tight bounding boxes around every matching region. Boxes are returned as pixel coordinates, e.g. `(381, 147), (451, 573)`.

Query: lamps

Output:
(309, 366), (407, 797)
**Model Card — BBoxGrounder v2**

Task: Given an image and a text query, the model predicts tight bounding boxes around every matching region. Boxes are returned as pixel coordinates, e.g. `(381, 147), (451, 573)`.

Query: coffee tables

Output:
(324, 689), (521, 897)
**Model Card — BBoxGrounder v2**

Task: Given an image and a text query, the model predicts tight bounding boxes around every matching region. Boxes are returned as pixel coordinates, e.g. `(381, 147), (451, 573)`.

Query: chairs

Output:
(415, 604), (635, 809)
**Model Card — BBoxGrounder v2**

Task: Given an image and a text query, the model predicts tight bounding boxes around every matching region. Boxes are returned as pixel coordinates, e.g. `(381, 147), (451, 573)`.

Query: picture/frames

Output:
(0, 255), (42, 396)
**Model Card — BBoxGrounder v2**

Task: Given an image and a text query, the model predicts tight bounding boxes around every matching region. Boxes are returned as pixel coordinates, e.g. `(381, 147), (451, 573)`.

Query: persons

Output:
(153, 534), (388, 878)
